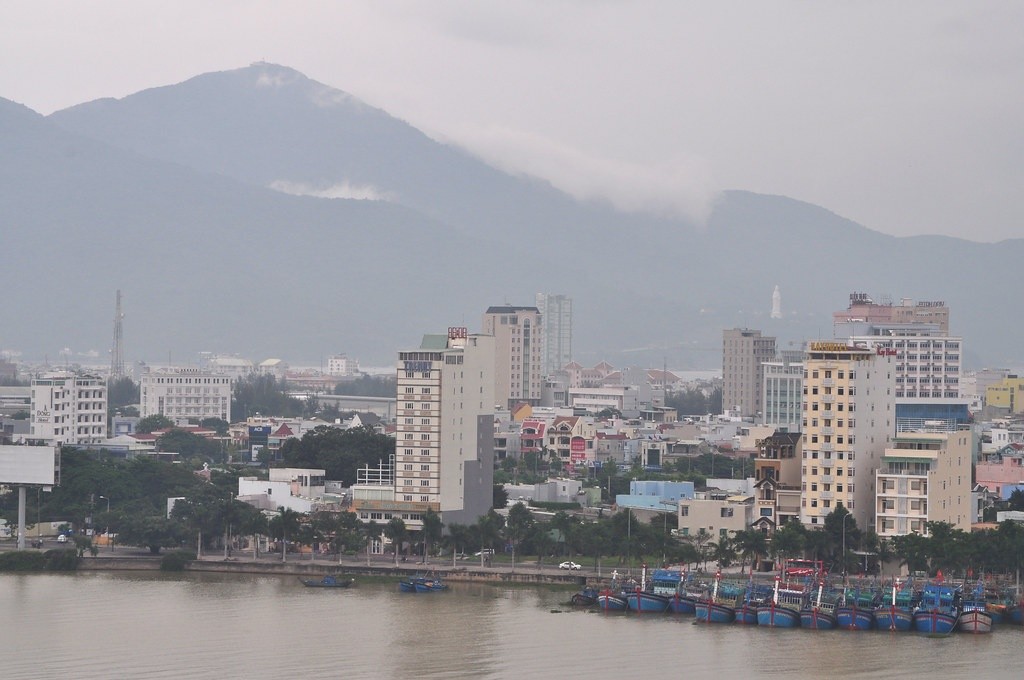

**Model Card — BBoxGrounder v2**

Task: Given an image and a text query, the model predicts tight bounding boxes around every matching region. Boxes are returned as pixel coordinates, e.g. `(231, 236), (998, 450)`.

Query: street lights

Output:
(100, 496), (110, 543)
(843, 512), (853, 575)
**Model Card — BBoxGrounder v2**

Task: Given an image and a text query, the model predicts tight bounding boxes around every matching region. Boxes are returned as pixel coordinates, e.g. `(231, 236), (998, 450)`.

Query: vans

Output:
(475, 549), (495, 556)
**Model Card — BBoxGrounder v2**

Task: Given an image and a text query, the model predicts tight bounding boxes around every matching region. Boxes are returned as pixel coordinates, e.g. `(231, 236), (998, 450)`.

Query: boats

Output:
(572, 561), (1024, 634)
(416, 555), (449, 591)
(298, 577), (354, 587)
(400, 563), (424, 590)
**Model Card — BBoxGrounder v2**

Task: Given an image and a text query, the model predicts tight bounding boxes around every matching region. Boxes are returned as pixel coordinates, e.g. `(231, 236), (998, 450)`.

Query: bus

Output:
(785, 558), (829, 577)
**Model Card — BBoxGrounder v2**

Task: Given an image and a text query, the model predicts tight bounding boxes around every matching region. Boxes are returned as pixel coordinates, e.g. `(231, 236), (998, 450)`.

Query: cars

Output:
(454, 554), (469, 561)
(559, 562), (581, 571)
